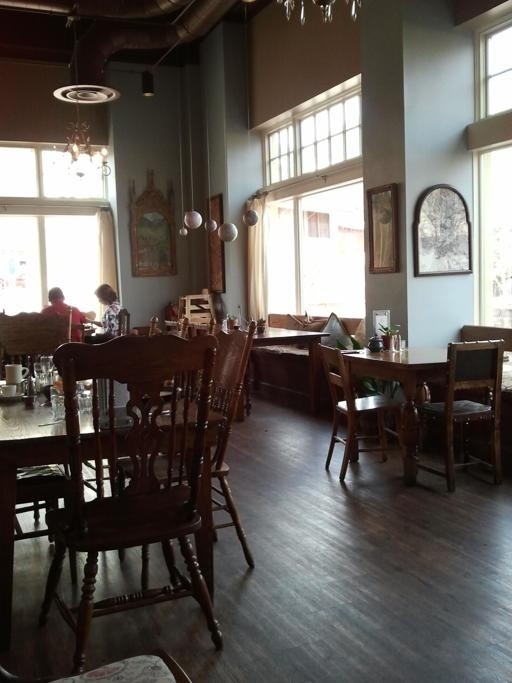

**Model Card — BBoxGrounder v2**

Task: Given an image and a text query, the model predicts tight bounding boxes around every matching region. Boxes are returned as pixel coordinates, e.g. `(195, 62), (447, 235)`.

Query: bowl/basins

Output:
(1, 385), (17, 397)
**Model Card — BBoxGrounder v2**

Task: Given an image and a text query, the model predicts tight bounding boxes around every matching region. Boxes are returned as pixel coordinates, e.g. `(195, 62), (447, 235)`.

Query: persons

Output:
(41, 287), (87, 344)
(83, 283), (127, 342)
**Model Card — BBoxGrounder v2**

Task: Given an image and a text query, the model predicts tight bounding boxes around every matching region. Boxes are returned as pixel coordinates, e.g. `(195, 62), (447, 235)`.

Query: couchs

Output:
(242, 313), (364, 414)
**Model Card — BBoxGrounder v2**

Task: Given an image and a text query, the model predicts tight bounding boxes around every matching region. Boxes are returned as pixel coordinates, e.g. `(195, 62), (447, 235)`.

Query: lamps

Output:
(50, 0), (110, 181)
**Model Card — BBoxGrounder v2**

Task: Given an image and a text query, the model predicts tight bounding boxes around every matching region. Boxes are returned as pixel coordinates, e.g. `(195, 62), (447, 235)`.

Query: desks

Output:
(228, 325), (330, 414)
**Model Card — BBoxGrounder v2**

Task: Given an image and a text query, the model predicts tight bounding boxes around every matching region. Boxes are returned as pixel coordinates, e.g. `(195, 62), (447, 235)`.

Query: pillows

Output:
(317, 312), (353, 349)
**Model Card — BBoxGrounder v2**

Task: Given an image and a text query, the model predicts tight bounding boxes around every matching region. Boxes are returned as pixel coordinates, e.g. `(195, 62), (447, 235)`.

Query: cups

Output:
(391, 334), (402, 352)
(4, 363), (30, 386)
(33, 354), (94, 420)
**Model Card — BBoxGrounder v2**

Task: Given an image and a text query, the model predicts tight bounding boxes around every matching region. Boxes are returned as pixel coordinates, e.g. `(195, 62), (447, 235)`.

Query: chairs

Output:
(316, 339), (504, 491)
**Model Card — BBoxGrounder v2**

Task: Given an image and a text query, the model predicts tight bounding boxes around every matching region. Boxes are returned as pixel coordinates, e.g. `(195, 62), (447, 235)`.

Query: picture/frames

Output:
(366, 182), (473, 276)
(127, 169), (225, 294)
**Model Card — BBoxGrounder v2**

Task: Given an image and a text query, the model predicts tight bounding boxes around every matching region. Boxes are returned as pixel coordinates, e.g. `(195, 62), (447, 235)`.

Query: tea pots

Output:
(364, 334), (384, 353)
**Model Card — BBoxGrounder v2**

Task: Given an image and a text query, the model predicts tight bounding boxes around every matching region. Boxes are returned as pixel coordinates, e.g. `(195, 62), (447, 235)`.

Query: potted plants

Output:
(378, 323), (400, 350)
